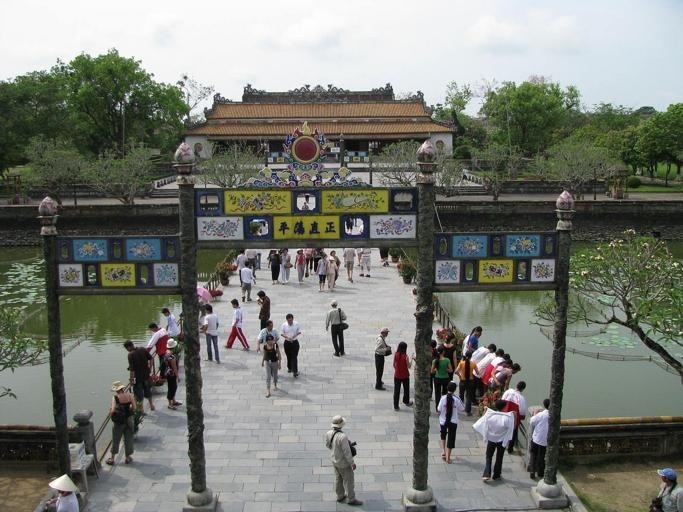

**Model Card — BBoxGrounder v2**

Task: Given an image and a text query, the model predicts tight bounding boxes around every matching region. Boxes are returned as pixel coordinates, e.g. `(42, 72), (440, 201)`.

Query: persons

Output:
(255, 312), (302, 398)
(654, 467), (683, 512)
(204, 304), (221, 363)
(325, 300), (348, 356)
(345, 218), (354, 234)
(326, 415), (363, 505)
(374, 325), (551, 483)
(304, 193), (310, 203)
(257, 290), (271, 330)
(302, 202), (310, 211)
(104, 307), (182, 465)
(359, 222), (364, 235)
(236, 246), (390, 303)
(45, 473), (80, 512)
(224, 299), (250, 351)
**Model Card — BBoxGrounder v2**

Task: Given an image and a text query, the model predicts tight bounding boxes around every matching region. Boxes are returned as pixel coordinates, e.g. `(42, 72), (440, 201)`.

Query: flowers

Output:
(436, 329), (447, 341)
(478, 390), (501, 415)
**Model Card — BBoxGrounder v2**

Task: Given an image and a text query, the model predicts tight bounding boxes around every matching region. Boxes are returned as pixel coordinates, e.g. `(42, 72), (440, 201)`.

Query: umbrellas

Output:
(197, 288), (213, 301)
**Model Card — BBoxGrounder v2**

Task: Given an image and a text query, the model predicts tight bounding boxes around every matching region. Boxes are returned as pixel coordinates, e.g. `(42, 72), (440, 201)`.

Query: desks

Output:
(69, 454), (99, 495)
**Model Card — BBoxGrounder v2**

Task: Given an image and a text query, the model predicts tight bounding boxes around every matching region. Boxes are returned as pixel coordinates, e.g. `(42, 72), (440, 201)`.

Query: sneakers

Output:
(125, 457), (133, 464)
(105, 457), (114, 465)
(492, 476), (501, 480)
(482, 476), (491, 480)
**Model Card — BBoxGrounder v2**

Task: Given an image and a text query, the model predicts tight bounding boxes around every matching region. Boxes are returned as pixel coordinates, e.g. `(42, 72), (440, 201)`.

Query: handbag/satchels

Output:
(350, 446), (357, 457)
(338, 323), (349, 330)
(110, 405), (125, 423)
(382, 346), (392, 356)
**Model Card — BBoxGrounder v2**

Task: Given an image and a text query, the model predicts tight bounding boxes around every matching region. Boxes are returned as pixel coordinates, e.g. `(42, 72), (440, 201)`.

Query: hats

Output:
(331, 300), (338, 306)
(111, 381), (126, 391)
(265, 334), (274, 340)
(380, 328), (391, 333)
(657, 467), (677, 481)
(166, 338), (178, 349)
(48, 473), (77, 492)
(330, 415), (346, 428)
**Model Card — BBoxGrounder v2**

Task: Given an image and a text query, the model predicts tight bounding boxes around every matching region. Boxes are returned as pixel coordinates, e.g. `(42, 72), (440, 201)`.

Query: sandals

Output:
(441, 452), (446, 459)
(173, 402), (183, 406)
(168, 405), (177, 410)
(446, 458), (451, 464)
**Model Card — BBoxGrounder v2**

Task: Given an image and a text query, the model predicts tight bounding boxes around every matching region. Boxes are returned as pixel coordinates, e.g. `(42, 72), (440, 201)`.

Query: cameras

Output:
(651, 496), (662, 511)
(348, 440), (357, 457)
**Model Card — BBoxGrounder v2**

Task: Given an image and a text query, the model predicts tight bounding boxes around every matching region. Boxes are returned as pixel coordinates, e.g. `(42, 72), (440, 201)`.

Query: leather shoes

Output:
(348, 499), (363, 506)
(336, 495), (346, 502)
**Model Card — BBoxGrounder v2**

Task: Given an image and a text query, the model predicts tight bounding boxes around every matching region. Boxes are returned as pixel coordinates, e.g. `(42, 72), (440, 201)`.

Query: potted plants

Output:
(388, 248), (405, 262)
(133, 402), (144, 434)
(398, 256), (416, 284)
(219, 269), (230, 285)
